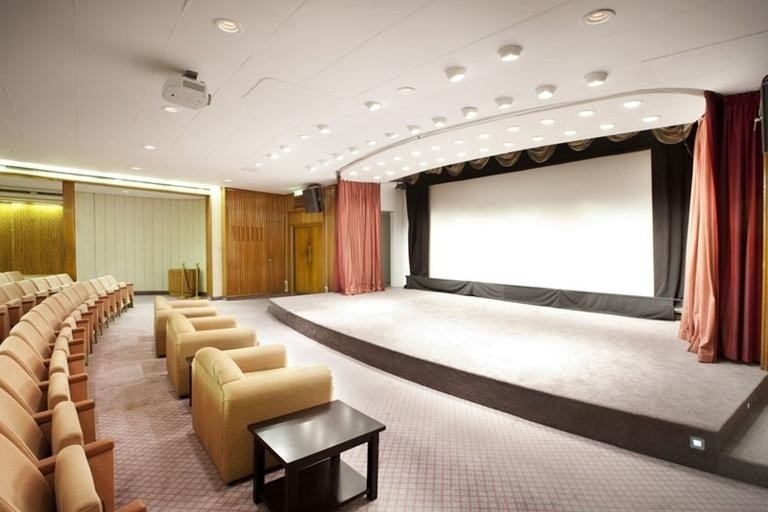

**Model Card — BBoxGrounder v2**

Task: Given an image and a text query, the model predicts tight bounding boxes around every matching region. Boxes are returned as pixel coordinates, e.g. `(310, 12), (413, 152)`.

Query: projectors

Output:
(160, 76), (212, 111)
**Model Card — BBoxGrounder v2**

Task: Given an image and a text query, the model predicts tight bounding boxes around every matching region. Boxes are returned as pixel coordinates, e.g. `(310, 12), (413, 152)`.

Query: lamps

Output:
(349, 145), (358, 153)
(464, 108), (478, 120)
(367, 102), (380, 112)
(497, 46), (523, 61)
(494, 97), (513, 109)
(319, 124), (330, 134)
(536, 85), (556, 99)
(407, 125), (422, 135)
(582, 71), (609, 87)
(433, 118), (445, 127)
(445, 66), (465, 83)
(334, 153), (344, 160)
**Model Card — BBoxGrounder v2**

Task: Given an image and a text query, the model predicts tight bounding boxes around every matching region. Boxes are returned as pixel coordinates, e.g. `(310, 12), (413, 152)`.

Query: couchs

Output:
(153, 295), (217, 356)
(192, 341), (333, 483)
(165, 314), (258, 397)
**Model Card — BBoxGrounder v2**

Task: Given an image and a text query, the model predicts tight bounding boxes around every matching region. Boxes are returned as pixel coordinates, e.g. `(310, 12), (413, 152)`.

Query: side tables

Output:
(247, 399), (385, 512)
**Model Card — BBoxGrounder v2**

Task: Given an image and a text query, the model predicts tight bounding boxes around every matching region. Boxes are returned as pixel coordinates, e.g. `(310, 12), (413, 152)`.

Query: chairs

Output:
(0, 271), (145, 512)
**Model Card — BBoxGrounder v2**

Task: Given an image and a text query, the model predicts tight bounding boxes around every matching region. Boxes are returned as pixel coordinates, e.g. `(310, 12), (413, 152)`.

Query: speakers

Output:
(303, 189), (318, 213)
